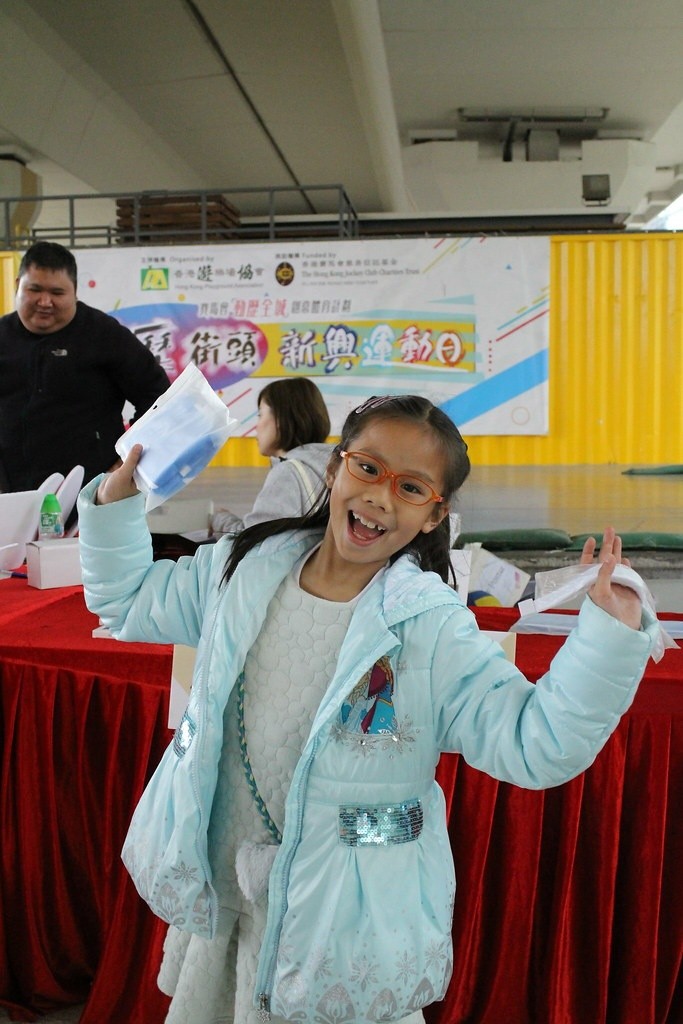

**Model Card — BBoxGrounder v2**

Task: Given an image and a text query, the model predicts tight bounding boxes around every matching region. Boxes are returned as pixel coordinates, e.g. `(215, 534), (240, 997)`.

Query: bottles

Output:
(39, 494), (64, 541)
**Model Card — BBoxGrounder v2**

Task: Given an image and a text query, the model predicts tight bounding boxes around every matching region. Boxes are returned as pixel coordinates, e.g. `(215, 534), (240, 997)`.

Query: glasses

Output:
(340, 450), (447, 503)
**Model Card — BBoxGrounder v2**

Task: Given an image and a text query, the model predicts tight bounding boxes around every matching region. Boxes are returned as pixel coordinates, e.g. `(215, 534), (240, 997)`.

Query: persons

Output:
(75, 395), (661, 1023)
(0, 242), (170, 528)
(208, 377), (339, 543)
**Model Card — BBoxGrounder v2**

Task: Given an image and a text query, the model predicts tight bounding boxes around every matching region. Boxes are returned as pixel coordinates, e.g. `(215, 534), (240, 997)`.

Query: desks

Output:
(0, 577), (683, 1024)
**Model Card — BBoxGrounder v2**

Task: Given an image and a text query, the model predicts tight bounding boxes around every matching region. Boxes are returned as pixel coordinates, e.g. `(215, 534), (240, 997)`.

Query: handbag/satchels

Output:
(235, 839), (284, 911)
